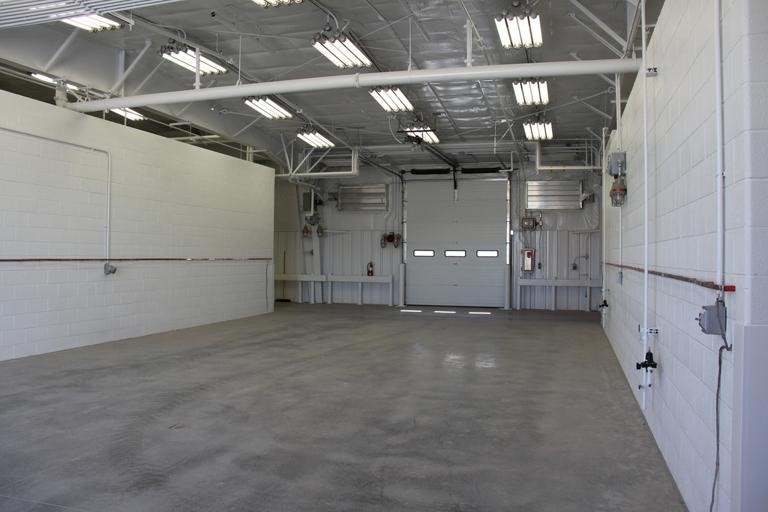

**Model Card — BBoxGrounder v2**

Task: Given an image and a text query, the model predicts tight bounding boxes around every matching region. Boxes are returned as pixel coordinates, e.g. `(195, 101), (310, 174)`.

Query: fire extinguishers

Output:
(367, 262), (373, 276)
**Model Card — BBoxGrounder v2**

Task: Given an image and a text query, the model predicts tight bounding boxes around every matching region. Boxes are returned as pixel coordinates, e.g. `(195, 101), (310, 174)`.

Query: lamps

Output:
(307, 13), (442, 146)
(252, 0), (302, 8)
(26, 0), (125, 33)
(158, 40), (228, 76)
(31, 73), (79, 92)
(495, 9), (554, 141)
(110, 106), (145, 122)
(241, 96), (293, 121)
(295, 129), (334, 149)
